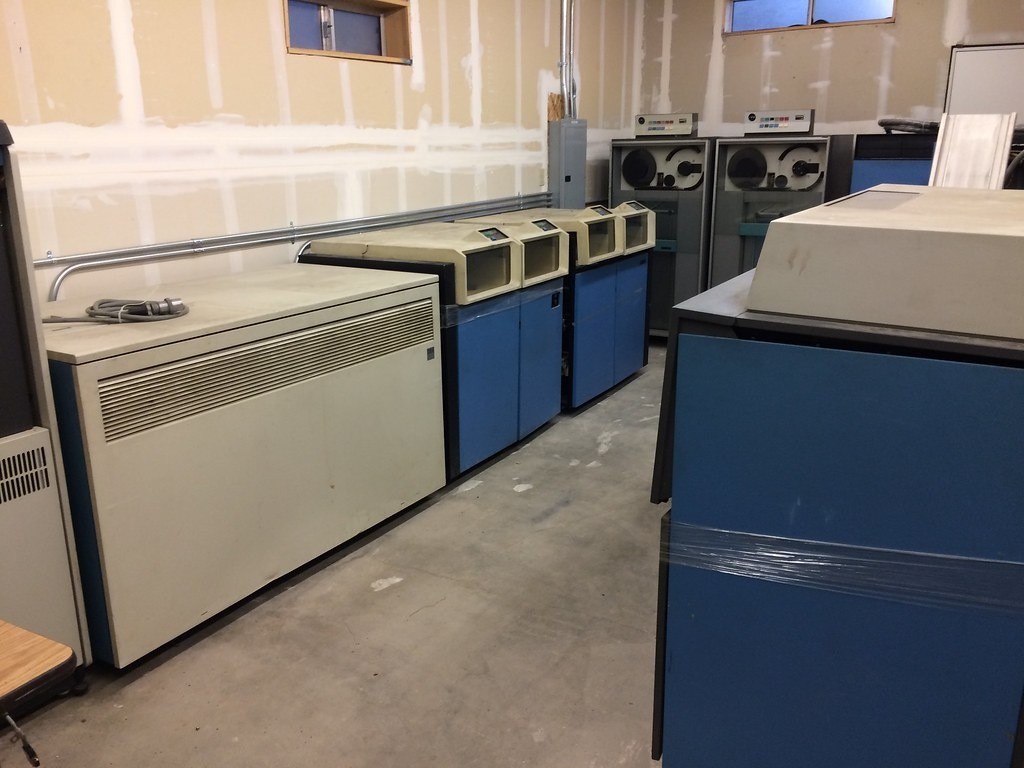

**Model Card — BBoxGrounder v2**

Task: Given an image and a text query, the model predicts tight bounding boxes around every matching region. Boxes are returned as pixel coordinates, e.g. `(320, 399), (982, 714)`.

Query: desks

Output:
(0, 619), (77, 767)
